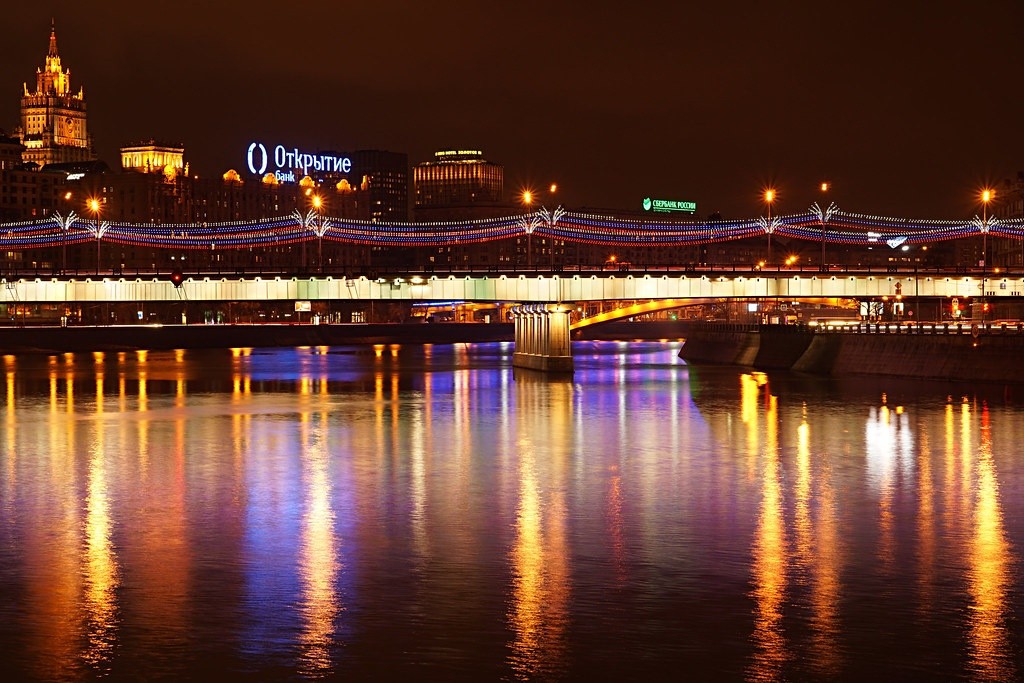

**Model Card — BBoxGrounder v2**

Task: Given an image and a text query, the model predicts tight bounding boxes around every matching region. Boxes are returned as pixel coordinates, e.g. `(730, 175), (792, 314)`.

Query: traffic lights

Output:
(983, 303), (989, 315)
(170, 267), (185, 288)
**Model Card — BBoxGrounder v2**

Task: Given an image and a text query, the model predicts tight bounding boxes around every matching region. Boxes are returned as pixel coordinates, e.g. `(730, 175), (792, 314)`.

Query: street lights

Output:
(86, 198), (101, 275)
(549, 183), (557, 270)
(764, 189), (775, 264)
(62, 191), (71, 269)
(820, 183), (828, 272)
(522, 191), (533, 270)
(312, 194), (323, 278)
(303, 188), (312, 270)
(980, 190), (991, 273)
